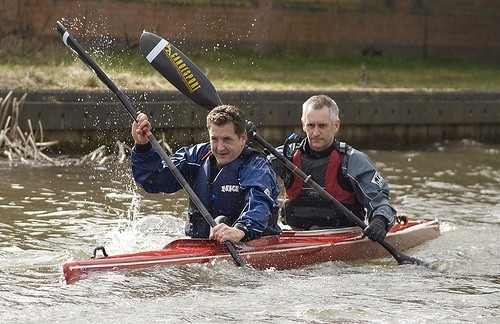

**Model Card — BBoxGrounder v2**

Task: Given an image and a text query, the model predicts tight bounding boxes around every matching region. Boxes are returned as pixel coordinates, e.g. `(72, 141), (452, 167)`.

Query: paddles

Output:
(139, 31), (436, 270)
(56, 20), (243, 266)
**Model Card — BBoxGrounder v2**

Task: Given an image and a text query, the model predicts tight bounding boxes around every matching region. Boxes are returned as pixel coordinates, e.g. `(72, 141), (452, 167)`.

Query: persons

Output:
(243, 94), (397, 243)
(131, 105), (282, 246)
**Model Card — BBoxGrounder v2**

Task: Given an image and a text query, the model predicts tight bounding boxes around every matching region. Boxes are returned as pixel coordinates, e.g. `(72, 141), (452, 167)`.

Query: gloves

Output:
(245, 120), (256, 136)
(362, 218), (386, 243)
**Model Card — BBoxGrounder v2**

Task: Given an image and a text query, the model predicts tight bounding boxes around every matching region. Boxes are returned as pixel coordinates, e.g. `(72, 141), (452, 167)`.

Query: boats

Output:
(61, 218), (439, 286)
